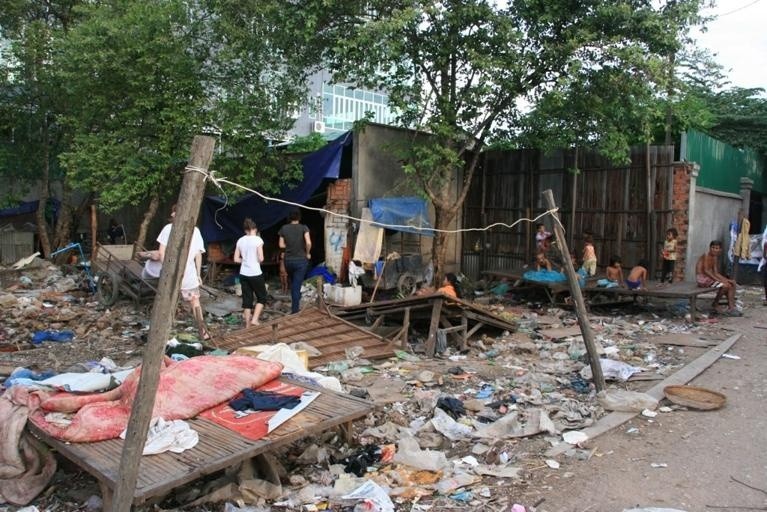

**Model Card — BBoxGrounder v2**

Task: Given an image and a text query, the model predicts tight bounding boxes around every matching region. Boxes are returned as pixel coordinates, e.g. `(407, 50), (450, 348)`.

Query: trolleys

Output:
(91, 241), (217, 312)
(361, 252), (433, 298)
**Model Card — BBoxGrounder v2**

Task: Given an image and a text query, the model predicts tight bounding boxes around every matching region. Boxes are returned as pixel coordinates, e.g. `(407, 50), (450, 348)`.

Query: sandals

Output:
(202, 330), (215, 340)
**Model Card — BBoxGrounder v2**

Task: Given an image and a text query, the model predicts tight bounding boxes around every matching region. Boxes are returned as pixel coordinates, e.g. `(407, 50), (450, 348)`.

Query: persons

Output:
(535, 240), (550, 272)
(596, 254), (624, 289)
(623, 258), (648, 289)
(755, 224), (766, 302)
(105, 218), (125, 244)
(433, 272), (461, 298)
(653, 227), (677, 287)
(534, 224), (553, 245)
(579, 235), (597, 276)
(276, 207), (313, 314)
(276, 251), (290, 296)
(231, 216), (267, 327)
(558, 247), (577, 274)
(694, 239), (744, 316)
(581, 228), (591, 242)
(155, 203), (214, 343)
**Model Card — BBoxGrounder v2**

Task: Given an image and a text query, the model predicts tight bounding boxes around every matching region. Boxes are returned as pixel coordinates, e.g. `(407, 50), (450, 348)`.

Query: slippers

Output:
(714, 310), (742, 317)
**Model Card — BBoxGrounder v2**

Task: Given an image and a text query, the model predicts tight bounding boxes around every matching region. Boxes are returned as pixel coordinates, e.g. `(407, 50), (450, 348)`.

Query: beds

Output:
(553, 277), (720, 322)
(27, 377), (380, 512)
